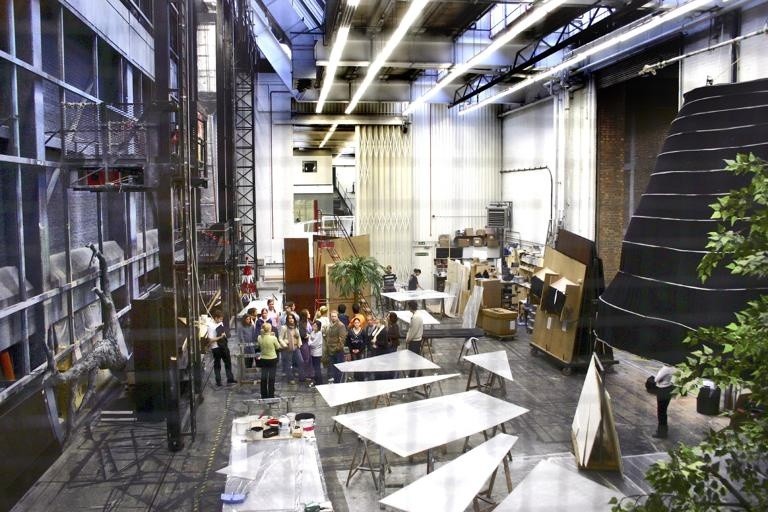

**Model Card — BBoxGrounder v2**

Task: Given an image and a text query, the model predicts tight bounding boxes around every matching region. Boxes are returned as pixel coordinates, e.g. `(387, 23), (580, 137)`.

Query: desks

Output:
(387, 306), (441, 361)
(461, 348), (514, 400)
(330, 350), (442, 400)
(479, 304), (519, 338)
(330, 387), (530, 492)
(310, 368), (463, 442)
(378, 431), (520, 511)
(487, 459), (644, 511)
(379, 284), (453, 313)
(220, 412), (336, 511)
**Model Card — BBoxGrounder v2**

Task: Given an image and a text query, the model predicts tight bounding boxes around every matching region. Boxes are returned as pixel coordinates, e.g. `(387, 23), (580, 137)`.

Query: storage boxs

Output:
(431, 228), (503, 248)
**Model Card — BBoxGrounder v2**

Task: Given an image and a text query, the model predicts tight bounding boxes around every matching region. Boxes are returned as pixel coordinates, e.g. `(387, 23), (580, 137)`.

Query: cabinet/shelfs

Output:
(433, 245), (545, 314)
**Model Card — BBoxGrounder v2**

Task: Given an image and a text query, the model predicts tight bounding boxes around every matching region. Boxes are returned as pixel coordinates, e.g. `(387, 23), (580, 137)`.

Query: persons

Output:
(381, 265), (397, 311)
(405, 300), (423, 379)
(237, 298), (400, 384)
(207, 310), (237, 386)
(257, 321), (279, 399)
(652, 362), (679, 440)
(407, 269), (425, 291)
